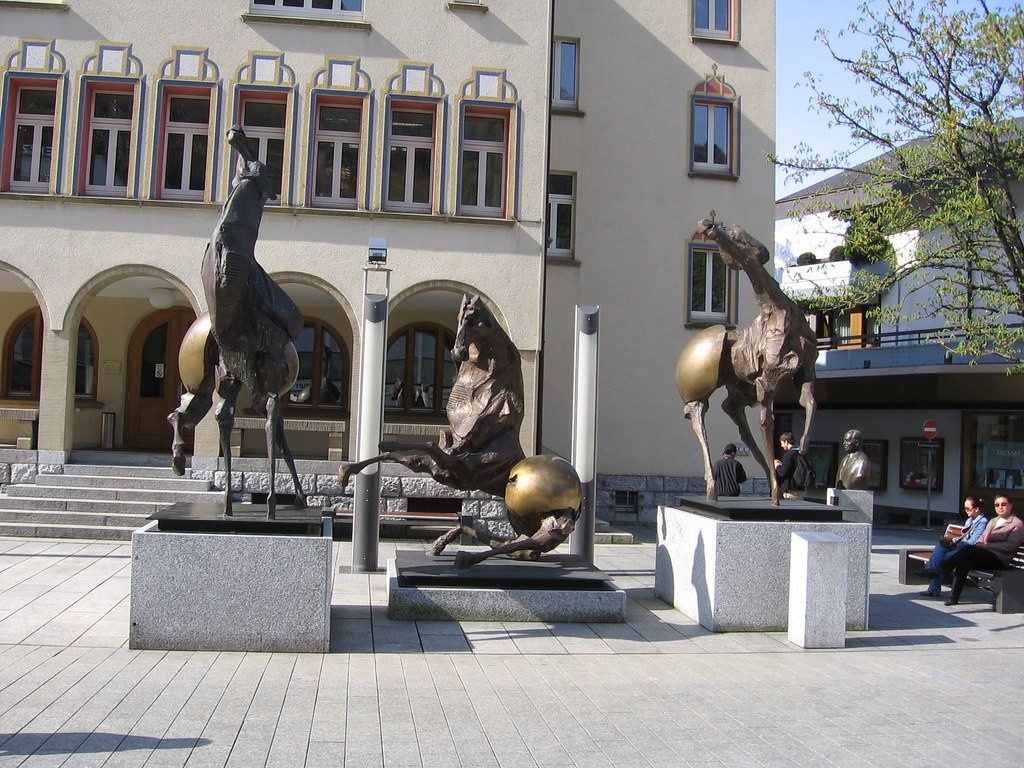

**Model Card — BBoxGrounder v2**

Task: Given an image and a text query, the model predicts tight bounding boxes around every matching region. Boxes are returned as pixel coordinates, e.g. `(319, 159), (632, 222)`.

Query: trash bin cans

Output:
(101, 412), (116, 451)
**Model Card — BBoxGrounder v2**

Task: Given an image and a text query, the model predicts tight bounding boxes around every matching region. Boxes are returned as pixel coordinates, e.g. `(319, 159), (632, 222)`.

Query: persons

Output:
(773, 431), (807, 502)
(835, 428), (871, 491)
(713, 443), (746, 497)
(917, 493), (1024, 606)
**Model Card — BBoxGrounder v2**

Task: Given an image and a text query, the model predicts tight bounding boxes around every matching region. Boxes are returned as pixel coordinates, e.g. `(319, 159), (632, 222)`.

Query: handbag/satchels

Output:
(939, 536), (958, 549)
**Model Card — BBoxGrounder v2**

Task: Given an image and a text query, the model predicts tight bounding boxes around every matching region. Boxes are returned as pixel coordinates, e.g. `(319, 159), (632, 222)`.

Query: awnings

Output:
(777, 261), (878, 309)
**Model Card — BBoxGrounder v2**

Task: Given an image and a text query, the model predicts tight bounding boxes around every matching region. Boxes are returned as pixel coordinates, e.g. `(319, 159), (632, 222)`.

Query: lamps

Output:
(150, 289), (176, 309)
(367, 237), (388, 267)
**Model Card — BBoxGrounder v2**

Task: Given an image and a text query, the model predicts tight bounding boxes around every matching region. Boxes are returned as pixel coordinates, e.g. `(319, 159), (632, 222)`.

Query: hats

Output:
(725, 444), (736, 452)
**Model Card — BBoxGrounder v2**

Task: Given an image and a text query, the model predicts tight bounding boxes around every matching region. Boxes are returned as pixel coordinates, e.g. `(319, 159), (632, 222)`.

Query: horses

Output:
(338, 293), (584, 572)
(675, 209), (820, 507)
(167, 123), (308, 522)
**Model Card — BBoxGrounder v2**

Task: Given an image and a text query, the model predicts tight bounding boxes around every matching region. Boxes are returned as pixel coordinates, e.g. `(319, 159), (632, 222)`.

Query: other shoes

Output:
(914, 569), (934, 577)
(944, 597), (958, 606)
(920, 590), (940, 596)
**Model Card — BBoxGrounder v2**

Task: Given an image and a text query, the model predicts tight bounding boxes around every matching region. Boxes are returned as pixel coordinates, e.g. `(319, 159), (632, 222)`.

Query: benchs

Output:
(899, 524), (1024, 613)
(322, 507), (474, 545)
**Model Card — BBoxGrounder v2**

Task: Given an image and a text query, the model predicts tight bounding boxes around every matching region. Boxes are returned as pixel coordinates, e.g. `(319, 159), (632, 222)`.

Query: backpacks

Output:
(787, 448), (816, 487)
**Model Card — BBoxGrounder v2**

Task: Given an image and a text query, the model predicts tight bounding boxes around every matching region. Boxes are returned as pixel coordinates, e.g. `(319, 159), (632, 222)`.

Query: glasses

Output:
(994, 503), (1008, 507)
(965, 506), (975, 512)
